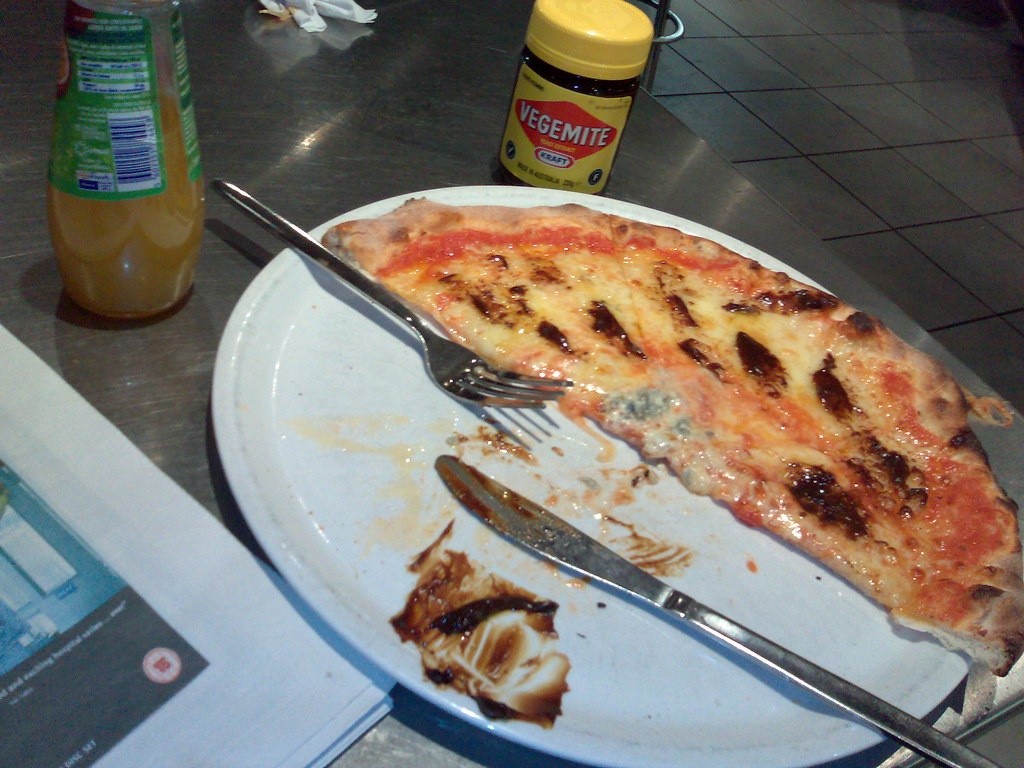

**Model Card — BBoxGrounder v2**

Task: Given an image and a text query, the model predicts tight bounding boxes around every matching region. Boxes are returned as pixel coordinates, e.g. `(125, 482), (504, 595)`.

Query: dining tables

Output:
(1, 0), (1024, 768)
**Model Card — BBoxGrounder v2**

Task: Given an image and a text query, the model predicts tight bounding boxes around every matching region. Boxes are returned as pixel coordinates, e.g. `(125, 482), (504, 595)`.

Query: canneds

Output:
(496, 0), (653, 197)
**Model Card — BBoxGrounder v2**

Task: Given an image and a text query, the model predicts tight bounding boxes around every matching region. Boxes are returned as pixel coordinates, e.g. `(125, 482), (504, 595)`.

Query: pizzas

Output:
(320, 195), (1024, 676)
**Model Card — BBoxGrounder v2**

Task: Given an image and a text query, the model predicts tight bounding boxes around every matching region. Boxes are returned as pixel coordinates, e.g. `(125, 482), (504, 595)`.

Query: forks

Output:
(211, 177), (574, 409)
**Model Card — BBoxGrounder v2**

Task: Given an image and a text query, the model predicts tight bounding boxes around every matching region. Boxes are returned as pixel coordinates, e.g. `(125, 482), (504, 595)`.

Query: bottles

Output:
(44, 1), (205, 320)
(498, 0), (662, 193)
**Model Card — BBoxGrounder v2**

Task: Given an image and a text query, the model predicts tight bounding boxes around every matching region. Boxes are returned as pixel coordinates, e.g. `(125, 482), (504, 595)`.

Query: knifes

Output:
(432, 458), (1010, 768)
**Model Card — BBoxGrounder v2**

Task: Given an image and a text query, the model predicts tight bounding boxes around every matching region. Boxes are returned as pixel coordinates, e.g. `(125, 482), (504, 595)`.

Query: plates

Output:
(211, 184), (1006, 768)
